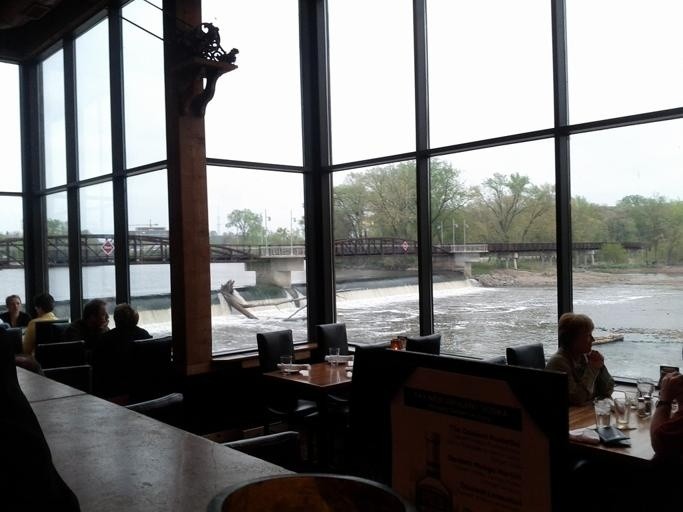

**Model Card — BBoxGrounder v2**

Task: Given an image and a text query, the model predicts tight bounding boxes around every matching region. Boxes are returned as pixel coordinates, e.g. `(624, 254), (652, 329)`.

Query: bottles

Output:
(636, 397), (645, 417)
(644, 396), (652, 416)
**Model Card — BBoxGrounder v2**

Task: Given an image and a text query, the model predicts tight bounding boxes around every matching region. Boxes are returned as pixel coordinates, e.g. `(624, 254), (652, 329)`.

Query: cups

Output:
(625, 390), (641, 410)
(328, 347), (340, 368)
(613, 396), (631, 429)
(280, 354), (292, 376)
(593, 400), (611, 428)
(636, 377), (657, 399)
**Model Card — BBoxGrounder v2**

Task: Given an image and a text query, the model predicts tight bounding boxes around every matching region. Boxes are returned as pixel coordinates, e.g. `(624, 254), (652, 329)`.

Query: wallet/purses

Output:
(594, 426), (632, 447)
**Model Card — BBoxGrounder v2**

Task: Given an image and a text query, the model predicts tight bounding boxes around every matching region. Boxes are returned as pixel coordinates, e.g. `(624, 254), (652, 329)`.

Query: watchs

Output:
(654, 399), (671, 408)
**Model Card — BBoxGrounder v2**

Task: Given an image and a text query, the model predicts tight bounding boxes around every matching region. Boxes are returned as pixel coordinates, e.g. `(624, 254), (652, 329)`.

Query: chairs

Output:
(481, 355), (504, 366)
(404, 333), (441, 355)
(221, 430), (306, 473)
(0, 318), (174, 404)
(256, 329), (318, 442)
(314, 323), (360, 409)
(328, 342), (392, 447)
(505, 343), (546, 369)
(125, 390), (183, 424)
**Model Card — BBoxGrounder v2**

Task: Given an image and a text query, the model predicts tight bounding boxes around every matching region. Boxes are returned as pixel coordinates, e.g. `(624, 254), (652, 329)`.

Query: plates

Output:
(330, 361), (347, 364)
(283, 368), (299, 373)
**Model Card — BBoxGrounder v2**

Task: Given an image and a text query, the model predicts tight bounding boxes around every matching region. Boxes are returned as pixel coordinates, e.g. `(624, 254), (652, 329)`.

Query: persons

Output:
(103, 303), (153, 340)
(545, 313), (613, 404)
(650, 371), (683, 454)
(62, 298), (111, 343)
(22, 291), (59, 355)
(0, 294), (31, 328)
